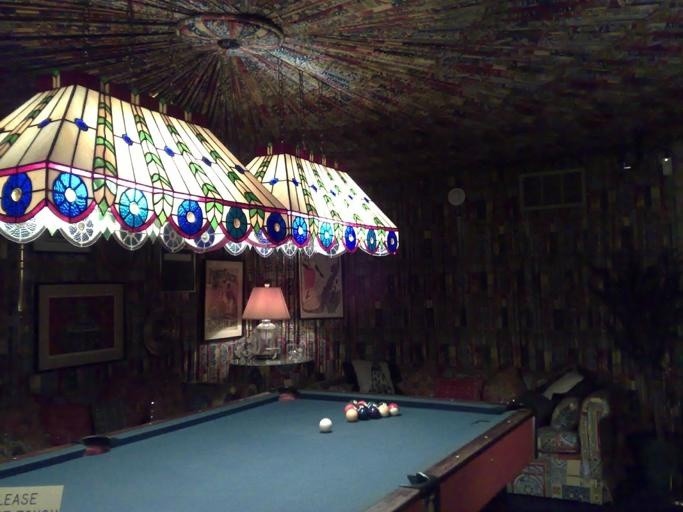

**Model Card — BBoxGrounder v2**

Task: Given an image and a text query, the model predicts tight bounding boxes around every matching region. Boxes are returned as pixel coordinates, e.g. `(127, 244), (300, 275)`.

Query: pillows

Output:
(105, 373), (150, 428)
(521, 376), (586, 427)
(351, 357), (401, 396)
(88, 397), (127, 435)
(397, 360), (436, 397)
(481, 367), (527, 402)
(39, 404), (94, 446)
(433, 377), (483, 400)
(551, 397), (580, 432)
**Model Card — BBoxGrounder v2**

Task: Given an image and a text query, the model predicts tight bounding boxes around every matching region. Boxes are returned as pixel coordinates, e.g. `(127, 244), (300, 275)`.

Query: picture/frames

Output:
(159, 247), (197, 294)
(298, 253), (345, 320)
(201, 258), (246, 344)
(35, 281), (126, 375)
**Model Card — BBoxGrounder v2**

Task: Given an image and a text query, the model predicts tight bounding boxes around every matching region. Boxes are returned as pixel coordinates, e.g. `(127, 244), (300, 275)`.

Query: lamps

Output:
(241, 282), (290, 360)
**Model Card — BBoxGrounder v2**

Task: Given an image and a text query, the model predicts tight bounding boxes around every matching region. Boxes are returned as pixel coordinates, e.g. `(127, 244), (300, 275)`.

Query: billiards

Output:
(344, 399), (399, 422)
(319, 418), (332, 432)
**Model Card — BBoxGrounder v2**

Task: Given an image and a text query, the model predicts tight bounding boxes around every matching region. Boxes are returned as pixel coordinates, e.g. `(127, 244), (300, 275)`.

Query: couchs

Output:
(306, 358), (623, 505)
(0, 381), (258, 459)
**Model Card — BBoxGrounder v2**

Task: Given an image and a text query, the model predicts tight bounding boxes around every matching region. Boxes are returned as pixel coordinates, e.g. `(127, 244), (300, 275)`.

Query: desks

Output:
(231, 356), (313, 390)
(0, 386), (538, 512)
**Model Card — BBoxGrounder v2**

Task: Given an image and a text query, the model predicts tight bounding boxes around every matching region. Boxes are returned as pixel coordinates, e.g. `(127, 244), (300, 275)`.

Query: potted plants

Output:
(586, 244), (683, 491)
